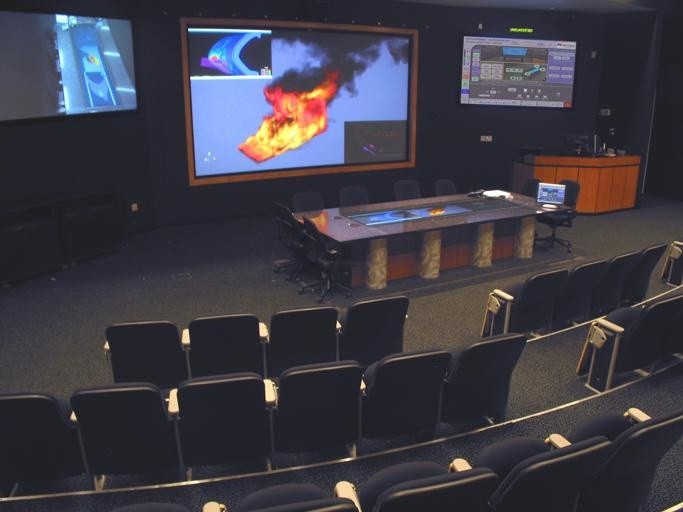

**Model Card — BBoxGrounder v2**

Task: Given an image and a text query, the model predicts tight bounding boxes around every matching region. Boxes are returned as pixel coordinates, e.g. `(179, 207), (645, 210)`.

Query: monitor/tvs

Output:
(536, 183), (567, 209)
(458, 33), (578, 111)
(0, 11), (142, 127)
(518, 145), (541, 160)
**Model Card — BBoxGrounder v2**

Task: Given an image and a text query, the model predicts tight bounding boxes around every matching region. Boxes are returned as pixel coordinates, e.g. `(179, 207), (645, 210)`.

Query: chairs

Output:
(338, 293), (412, 364)
(259, 177), (584, 303)
(101, 319), (184, 387)
(3, 390), (93, 495)
(256, 305), (339, 374)
(422, 330), (530, 430)
(446, 431), (616, 511)
(264, 359), (364, 464)
(179, 373), (281, 481)
(475, 240), (681, 395)
(570, 405), (682, 510)
(179, 312), (261, 377)
(357, 345), (453, 448)
(331, 459), (500, 511)
(70, 380), (180, 494)
(196, 479), (355, 511)
